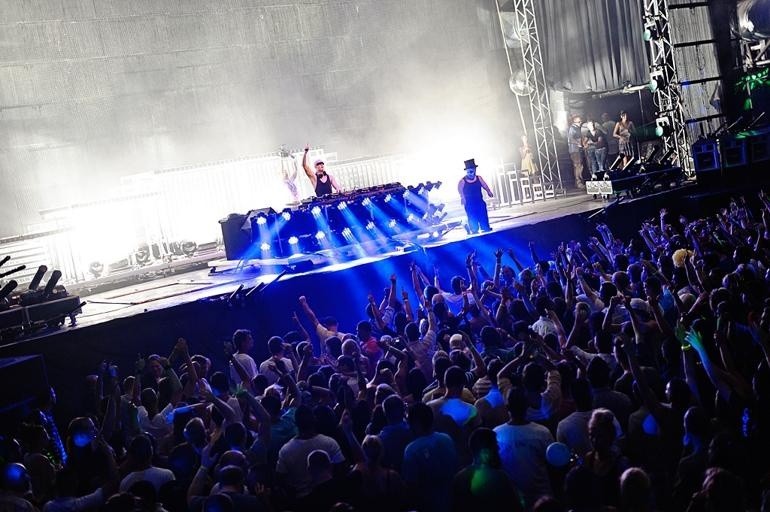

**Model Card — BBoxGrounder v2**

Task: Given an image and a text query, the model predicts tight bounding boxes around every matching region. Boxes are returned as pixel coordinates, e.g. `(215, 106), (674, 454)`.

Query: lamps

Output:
(640, 14), (679, 138)
(255, 180), (445, 253)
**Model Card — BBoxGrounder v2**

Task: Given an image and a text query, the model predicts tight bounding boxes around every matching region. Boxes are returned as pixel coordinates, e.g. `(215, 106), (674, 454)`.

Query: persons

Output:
(566, 109), (641, 191)
(411, 190), (768, 509)
(301, 141), (344, 198)
(518, 135), (540, 187)
(457, 158), (495, 234)
(2, 276), (518, 510)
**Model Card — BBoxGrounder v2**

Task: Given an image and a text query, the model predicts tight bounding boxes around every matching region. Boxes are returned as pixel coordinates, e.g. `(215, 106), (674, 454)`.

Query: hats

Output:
(619, 298), (650, 313)
(314, 160), (324, 167)
(463, 159), (478, 170)
